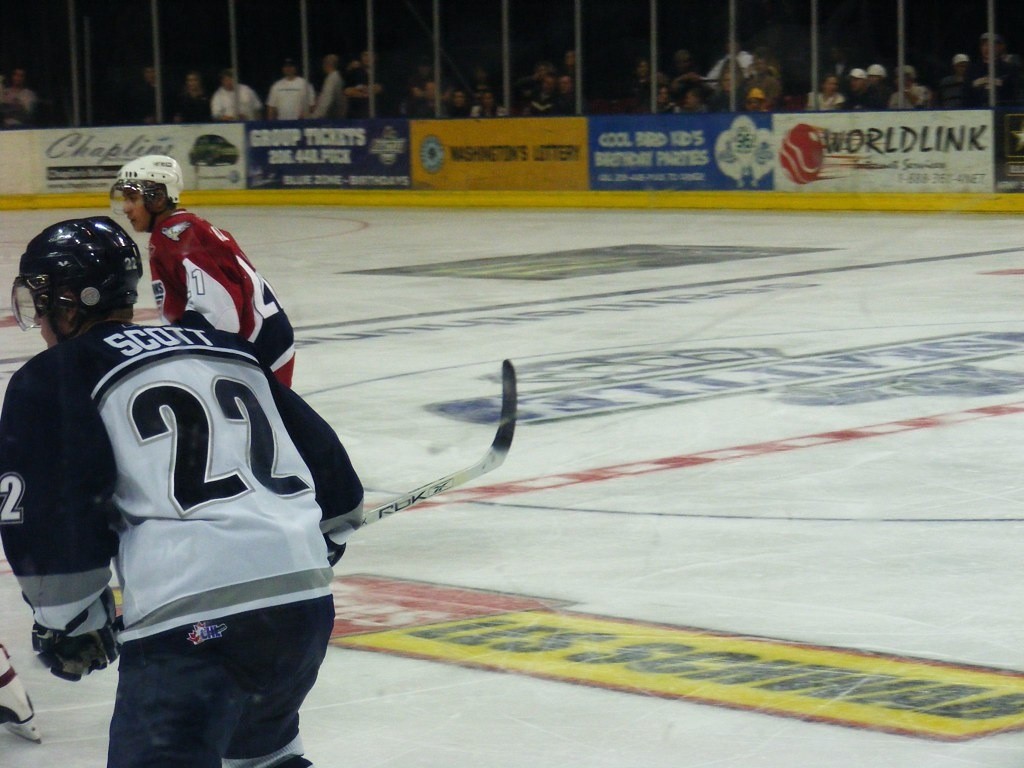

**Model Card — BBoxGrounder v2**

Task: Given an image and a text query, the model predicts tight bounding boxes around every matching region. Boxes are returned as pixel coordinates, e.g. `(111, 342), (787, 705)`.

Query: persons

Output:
(139, 23), (1024, 124)
(0, 214), (367, 768)
(0, 644), (41, 745)
(111, 152), (294, 384)
(0, 64), (41, 130)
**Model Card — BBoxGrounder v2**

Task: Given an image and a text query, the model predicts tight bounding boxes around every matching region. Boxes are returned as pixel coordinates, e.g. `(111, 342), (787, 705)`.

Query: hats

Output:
(737, 51), (754, 68)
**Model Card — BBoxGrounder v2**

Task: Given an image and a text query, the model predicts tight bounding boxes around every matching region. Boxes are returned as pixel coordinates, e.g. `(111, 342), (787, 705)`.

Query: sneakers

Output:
(0, 642), (43, 744)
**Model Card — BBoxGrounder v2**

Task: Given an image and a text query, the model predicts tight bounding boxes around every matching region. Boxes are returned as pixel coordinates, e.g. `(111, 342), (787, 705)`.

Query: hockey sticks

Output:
(27, 358), (519, 672)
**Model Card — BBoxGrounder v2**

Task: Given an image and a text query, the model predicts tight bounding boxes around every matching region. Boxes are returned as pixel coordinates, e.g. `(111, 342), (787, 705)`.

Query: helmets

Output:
(747, 88), (765, 99)
(114, 155), (185, 203)
(979, 32), (998, 40)
(849, 68), (867, 79)
(866, 64), (886, 75)
(18, 216), (143, 322)
(952, 54), (969, 66)
(896, 65), (915, 79)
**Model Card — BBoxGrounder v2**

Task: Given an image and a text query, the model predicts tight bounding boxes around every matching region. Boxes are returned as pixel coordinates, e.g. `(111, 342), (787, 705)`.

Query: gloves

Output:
(31, 621), (122, 681)
